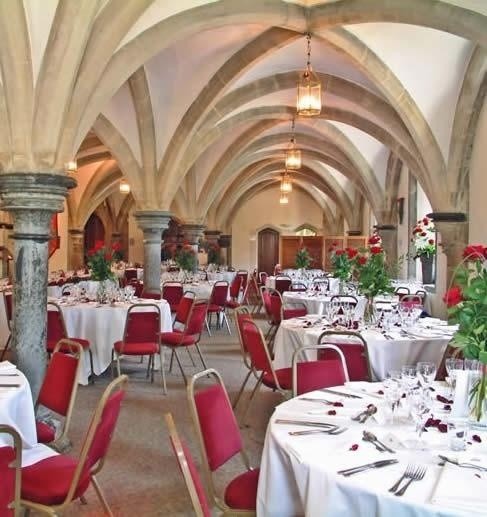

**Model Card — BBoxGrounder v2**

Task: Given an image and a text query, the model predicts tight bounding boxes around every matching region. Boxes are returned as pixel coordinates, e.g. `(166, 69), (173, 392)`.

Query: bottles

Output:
(451, 372), (470, 417)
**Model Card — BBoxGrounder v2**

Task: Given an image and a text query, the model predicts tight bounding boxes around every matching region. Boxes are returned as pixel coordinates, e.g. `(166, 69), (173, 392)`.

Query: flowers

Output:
(328, 241), (366, 279)
(295, 240), (312, 267)
(409, 219), (436, 260)
(351, 235), (395, 298)
(175, 240), (198, 268)
(440, 244), (487, 372)
(87, 240), (122, 278)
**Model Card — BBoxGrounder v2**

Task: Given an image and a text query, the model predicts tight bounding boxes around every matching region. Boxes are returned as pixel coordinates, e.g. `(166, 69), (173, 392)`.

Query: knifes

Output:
(275, 422), (331, 429)
(439, 454), (487, 472)
(320, 388), (362, 398)
(343, 461), (399, 477)
(337, 459), (397, 475)
(275, 419), (336, 426)
(362, 436), (385, 452)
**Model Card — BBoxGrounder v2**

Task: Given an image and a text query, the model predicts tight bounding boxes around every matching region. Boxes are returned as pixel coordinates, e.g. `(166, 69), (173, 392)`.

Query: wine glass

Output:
(445, 358), (464, 392)
(47, 256), (138, 302)
(308, 281), (359, 295)
(417, 361), (437, 398)
(403, 365), (417, 396)
(384, 371), (425, 449)
(161, 269), (212, 285)
(283, 268), (324, 281)
(465, 359), (480, 381)
(324, 297), (418, 332)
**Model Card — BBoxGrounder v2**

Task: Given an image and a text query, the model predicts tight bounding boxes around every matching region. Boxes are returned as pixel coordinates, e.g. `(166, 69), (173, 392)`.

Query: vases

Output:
(298, 267), (307, 280)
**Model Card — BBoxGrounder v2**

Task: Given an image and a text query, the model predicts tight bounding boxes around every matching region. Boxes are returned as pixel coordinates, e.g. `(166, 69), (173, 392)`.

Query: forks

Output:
(395, 466), (428, 497)
(388, 463), (415, 492)
(299, 398), (343, 404)
(289, 428), (349, 435)
(288, 426), (339, 434)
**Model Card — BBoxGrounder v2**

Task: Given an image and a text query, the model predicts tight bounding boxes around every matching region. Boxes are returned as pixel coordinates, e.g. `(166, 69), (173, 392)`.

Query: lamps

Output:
(296, 32), (321, 117)
(278, 171), (292, 207)
(119, 178), (131, 194)
(67, 157), (78, 174)
(285, 112), (301, 170)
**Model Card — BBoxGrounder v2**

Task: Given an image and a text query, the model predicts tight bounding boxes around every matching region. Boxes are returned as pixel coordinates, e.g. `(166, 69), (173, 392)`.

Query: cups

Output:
(447, 421), (470, 452)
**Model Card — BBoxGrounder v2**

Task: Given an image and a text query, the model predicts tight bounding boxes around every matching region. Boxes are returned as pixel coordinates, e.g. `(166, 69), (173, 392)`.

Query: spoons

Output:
(363, 430), (396, 454)
(352, 411), (366, 421)
(360, 407), (378, 423)
